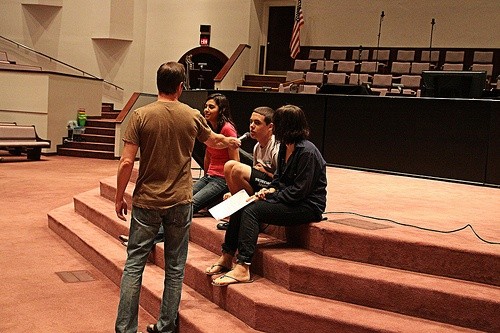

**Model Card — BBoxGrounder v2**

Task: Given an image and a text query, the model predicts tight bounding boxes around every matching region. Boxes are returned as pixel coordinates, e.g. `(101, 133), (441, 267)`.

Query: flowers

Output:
(66, 120), (78, 130)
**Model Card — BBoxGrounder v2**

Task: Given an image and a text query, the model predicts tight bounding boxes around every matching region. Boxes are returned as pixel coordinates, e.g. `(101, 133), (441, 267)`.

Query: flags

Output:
(289, 0), (304, 59)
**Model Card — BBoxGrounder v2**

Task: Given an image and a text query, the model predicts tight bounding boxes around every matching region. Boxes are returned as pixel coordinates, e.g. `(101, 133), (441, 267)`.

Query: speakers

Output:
(420, 70), (487, 99)
(319, 83), (369, 95)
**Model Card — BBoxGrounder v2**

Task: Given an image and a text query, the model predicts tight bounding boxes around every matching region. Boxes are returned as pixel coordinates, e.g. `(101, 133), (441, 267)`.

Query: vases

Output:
(68, 129), (73, 140)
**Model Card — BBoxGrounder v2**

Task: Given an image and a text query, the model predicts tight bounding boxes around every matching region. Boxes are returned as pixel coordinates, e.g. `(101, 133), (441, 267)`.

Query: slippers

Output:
(211, 275), (253, 287)
(205, 263), (233, 275)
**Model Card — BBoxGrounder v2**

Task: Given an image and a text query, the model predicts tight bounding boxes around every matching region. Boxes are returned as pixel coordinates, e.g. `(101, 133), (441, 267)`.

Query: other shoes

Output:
(120, 233), (128, 246)
(147, 324), (160, 333)
(217, 221), (229, 230)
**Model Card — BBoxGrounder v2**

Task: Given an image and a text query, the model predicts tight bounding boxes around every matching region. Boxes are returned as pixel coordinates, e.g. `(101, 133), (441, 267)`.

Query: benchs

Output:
(0, 121), (51, 162)
(0, 51), (16, 64)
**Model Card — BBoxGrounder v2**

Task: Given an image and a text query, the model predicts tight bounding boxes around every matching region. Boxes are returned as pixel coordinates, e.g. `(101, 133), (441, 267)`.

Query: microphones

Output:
(381, 11), (385, 19)
(238, 132), (250, 141)
(432, 18), (435, 25)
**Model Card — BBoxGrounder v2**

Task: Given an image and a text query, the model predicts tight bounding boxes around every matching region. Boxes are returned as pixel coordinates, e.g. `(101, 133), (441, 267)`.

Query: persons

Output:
(206, 105), (326, 286)
(114, 61), (241, 333)
(119, 94), (240, 248)
(217, 107), (281, 229)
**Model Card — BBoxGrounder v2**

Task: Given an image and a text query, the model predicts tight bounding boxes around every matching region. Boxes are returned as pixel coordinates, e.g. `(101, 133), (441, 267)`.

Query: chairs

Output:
(279, 48), (494, 94)
